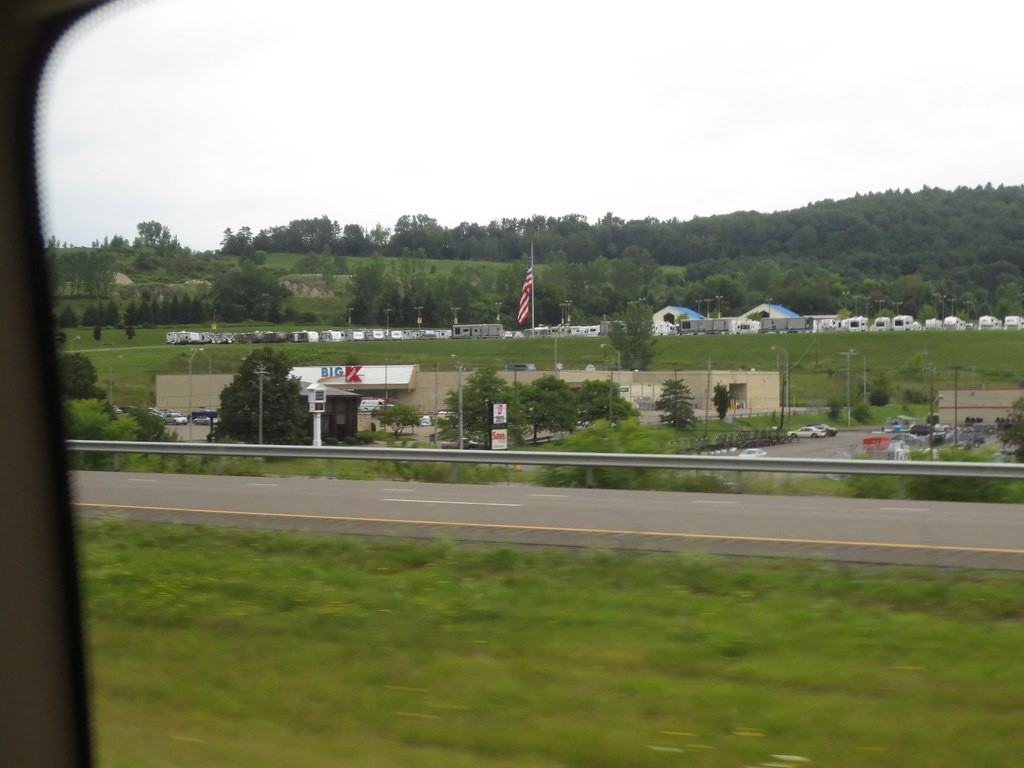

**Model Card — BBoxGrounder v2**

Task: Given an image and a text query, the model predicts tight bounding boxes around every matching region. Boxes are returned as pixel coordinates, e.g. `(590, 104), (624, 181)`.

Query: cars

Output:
(787, 426), (825, 438)
(806, 423), (839, 437)
(441, 437), (486, 450)
(112, 403), (182, 423)
(418, 411), (456, 427)
(162, 413), (188, 425)
(860, 415), (987, 451)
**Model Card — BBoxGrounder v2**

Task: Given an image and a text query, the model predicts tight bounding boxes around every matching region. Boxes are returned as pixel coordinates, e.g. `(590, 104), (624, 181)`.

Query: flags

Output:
(517, 248), (532, 325)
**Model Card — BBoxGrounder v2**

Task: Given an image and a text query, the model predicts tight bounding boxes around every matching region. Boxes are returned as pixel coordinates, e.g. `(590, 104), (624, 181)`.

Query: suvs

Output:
(360, 399), (396, 413)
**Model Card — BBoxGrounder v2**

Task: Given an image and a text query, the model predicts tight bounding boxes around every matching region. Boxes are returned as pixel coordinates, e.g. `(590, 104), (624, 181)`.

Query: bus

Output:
(190, 410), (219, 426)
